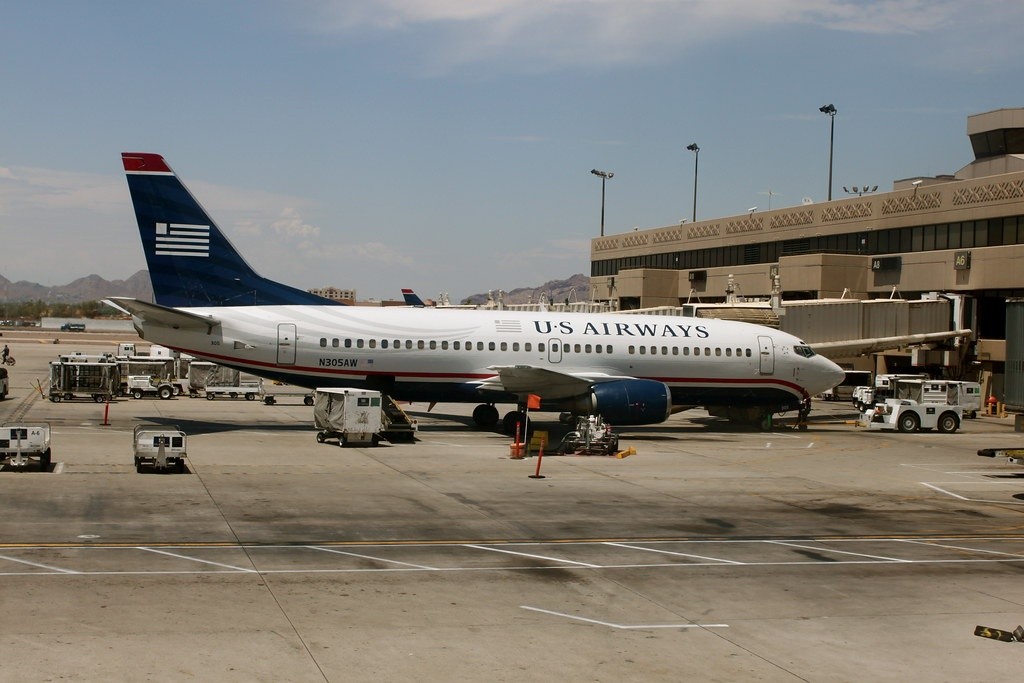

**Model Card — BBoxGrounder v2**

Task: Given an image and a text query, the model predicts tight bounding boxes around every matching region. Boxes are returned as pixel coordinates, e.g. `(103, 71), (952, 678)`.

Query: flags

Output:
(527, 394), (541, 409)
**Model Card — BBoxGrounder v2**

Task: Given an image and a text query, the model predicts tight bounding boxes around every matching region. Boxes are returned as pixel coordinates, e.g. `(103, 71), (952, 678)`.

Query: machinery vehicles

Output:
(126, 373), (174, 400)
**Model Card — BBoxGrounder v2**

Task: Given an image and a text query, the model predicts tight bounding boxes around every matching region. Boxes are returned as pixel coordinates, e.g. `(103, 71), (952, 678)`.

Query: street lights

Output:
(687, 143), (699, 222)
(591, 169), (614, 237)
(819, 103), (837, 202)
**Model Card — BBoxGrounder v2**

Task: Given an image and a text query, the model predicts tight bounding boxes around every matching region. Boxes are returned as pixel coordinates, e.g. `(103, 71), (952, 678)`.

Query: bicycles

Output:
(0, 353), (16, 366)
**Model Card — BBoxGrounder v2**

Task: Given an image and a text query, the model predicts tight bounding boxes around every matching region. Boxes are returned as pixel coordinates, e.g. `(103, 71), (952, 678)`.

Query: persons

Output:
(0, 345), (9, 364)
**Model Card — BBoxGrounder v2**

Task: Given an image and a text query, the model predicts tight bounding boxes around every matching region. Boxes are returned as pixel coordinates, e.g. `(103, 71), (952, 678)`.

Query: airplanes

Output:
(97, 151), (846, 441)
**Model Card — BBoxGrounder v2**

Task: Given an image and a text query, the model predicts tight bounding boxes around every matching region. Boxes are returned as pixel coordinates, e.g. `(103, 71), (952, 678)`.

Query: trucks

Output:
(0, 368), (10, 402)
(821, 370), (981, 433)
